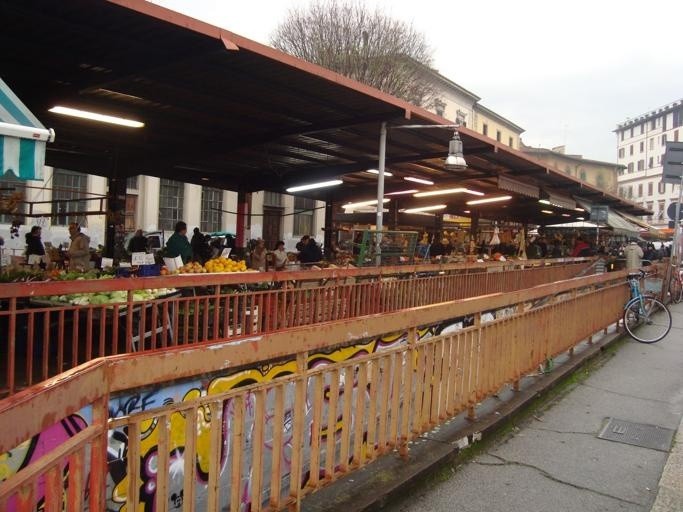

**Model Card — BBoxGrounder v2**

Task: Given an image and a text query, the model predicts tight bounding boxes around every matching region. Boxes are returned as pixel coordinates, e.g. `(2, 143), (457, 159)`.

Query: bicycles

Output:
(621, 268), (672, 345)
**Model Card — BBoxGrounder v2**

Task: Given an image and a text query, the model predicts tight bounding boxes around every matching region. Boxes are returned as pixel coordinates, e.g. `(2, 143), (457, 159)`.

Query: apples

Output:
(160, 261), (207, 275)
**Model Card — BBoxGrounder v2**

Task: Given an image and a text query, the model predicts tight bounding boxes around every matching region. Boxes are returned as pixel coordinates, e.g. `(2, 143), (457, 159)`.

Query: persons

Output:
(525, 229), (672, 269)
(65, 222), (91, 273)
(127, 229), (150, 255)
(166, 220), (193, 267)
(191, 228), (236, 267)
(248, 236), (269, 270)
(26, 225), (50, 272)
(502, 241), (519, 257)
(269, 241), (289, 288)
(379, 228), (482, 264)
(296, 233), (374, 267)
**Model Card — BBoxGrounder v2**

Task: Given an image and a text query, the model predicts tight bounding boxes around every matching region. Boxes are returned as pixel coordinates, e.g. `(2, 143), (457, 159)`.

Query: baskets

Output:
(644, 278), (664, 293)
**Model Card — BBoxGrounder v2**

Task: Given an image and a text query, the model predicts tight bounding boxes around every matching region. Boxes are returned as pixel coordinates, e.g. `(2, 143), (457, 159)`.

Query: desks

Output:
(27, 291), (184, 354)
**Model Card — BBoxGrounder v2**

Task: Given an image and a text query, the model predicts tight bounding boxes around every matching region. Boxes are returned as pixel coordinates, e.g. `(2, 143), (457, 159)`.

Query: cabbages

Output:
(31, 272), (155, 308)
